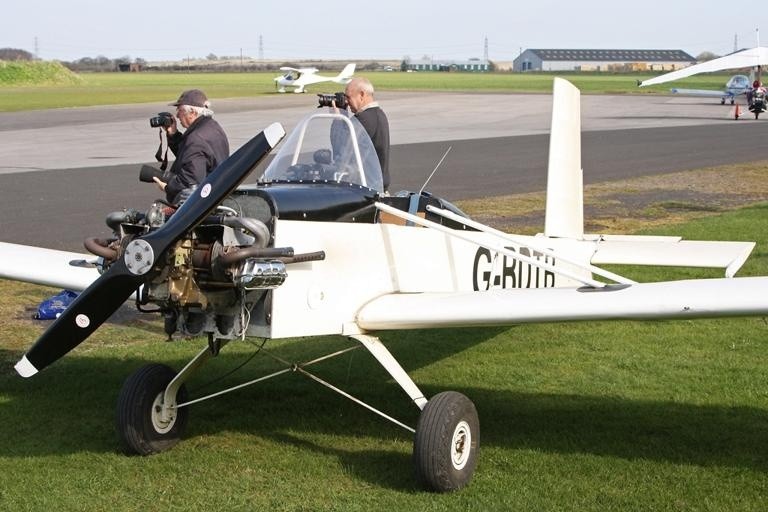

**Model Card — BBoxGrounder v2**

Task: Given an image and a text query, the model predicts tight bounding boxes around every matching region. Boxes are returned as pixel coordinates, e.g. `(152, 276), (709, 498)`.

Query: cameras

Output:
(150, 112), (173, 127)
(317, 93), (346, 109)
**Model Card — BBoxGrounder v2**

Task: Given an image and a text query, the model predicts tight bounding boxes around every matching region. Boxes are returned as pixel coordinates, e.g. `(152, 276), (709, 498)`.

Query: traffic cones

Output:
(734, 103), (742, 120)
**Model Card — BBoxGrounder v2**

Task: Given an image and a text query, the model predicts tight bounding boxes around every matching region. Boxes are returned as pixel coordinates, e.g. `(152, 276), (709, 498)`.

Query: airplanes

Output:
(273, 62), (357, 94)
(1, 73), (768, 496)
(669, 69), (768, 106)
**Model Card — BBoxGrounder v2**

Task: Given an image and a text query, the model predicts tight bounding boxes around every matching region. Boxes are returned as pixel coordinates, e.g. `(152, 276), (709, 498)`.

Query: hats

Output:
(168, 90), (208, 109)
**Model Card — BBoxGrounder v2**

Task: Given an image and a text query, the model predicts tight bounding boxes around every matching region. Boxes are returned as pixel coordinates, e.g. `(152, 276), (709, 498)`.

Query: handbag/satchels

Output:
(35, 289), (83, 319)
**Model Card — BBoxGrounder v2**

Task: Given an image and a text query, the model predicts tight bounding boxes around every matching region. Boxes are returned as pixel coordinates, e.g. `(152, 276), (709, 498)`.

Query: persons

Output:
(152, 87), (230, 206)
(328, 76), (392, 191)
